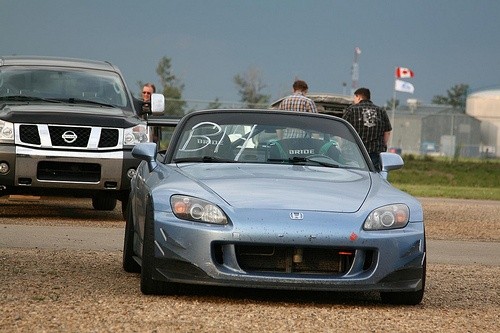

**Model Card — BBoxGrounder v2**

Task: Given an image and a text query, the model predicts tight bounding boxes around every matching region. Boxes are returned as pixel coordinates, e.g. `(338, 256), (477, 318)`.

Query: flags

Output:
(395, 79), (414, 93)
(393, 65), (414, 79)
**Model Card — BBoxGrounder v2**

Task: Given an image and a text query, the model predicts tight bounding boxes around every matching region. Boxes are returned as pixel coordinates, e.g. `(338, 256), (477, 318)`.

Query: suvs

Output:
(0, 57), (148, 211)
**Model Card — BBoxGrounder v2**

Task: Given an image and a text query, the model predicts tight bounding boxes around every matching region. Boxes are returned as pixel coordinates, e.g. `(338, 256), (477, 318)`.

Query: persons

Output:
(276, 80), (320, 139)
(342, 88), (392, 173)
(139, 83), (162, 144)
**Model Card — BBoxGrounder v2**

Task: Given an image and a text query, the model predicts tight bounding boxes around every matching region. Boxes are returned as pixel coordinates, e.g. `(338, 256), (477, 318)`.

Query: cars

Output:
(145, 116), (178, 159)
(269, 95), (356, 119)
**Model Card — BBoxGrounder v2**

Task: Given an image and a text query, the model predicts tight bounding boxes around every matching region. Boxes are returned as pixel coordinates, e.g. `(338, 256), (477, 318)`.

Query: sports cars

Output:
(120, 107), (423, 305)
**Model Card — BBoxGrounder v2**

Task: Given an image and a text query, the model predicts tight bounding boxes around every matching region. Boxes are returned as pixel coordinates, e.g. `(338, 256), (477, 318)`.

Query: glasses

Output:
(142, 92), (151, 94)
